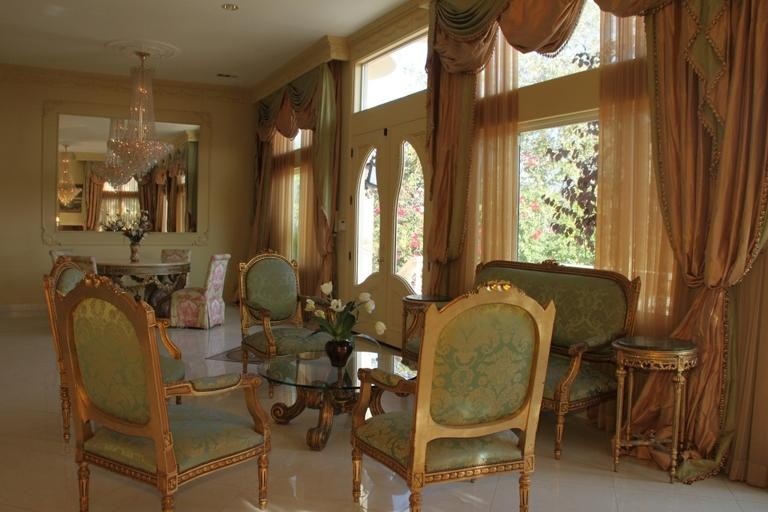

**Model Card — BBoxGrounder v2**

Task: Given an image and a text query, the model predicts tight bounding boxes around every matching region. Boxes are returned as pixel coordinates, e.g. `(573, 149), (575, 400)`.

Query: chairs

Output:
(351, 280), (555, 512)
(171, 254), (230, 330)
(57, 275), (268, 512)
(473, 261), (641, 457)
(238, 249), (334, 401)
(45, 256), (182, 446)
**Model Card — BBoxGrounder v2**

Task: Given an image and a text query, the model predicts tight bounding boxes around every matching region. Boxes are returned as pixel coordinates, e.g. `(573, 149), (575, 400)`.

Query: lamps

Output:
(108, 52), (173, 179)
(59, 144), (79, 207)
(104, 118), (133, 188)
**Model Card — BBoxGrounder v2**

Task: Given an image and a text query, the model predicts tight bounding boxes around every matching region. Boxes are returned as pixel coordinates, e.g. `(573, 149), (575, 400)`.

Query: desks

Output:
(258, 352), (419, 450)
(96, 260), (190, 314)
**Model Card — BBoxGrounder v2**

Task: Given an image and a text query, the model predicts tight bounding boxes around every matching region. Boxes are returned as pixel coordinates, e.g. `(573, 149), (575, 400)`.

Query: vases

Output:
(131, 244), (140, 265)
(327, 339), (353, 367)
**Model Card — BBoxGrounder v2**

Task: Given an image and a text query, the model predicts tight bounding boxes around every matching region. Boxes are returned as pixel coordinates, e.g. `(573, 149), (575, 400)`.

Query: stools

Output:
(402, 294), (454, 360)
(610, 337), (700, 483)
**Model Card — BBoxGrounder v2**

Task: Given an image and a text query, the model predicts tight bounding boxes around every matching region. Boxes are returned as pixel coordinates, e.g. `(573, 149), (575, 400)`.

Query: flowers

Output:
(104, 211), (150, 243)
(297, 282), (386, 348)
